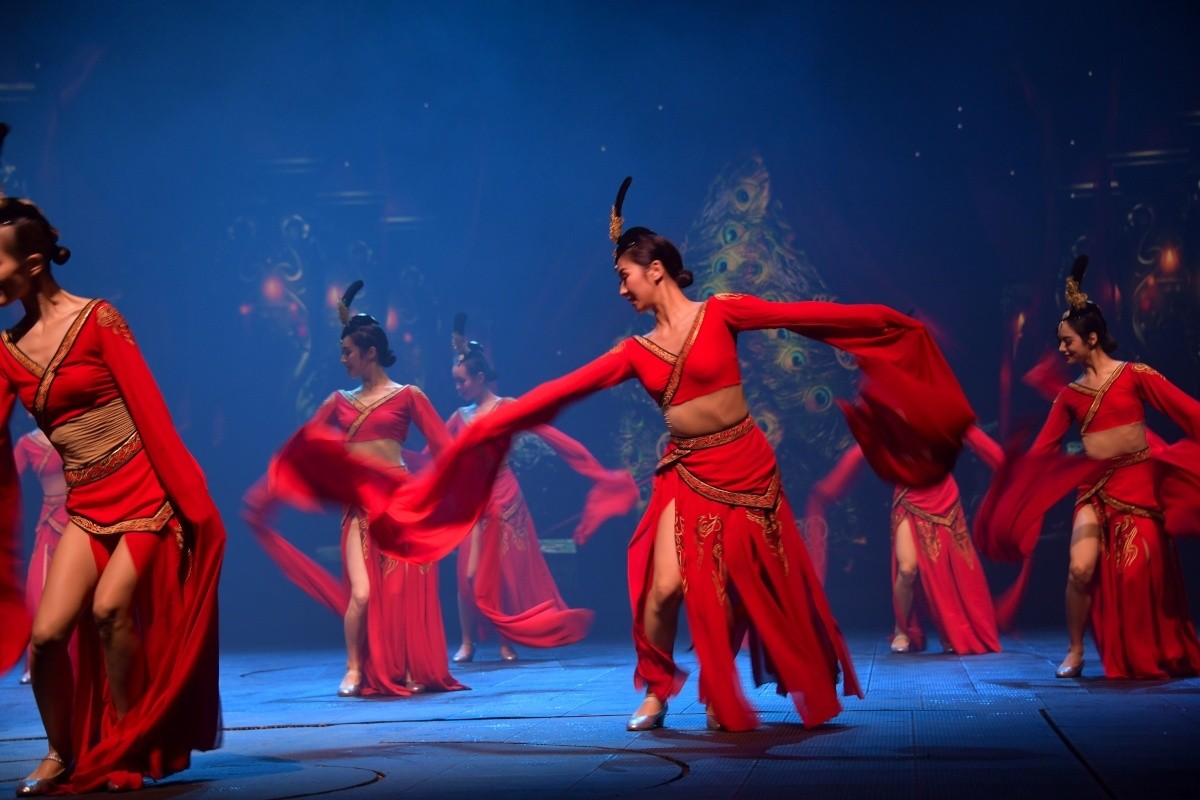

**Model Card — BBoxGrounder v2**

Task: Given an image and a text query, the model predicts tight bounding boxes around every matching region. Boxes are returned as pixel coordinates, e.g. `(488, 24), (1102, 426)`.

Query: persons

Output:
(239, 313), (621, 698)
(0, 196), (229, 800)
(13, 407), (70, 684)
(435, 224), (928, 733)
(813, 422), (1009, 656)
(993, 298), (1200, 682)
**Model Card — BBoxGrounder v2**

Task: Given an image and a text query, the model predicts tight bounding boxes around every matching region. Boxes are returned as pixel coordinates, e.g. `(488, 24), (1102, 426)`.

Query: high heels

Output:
(14, 752), (70, 798)
(500, 640), (518, 662)
(626, 687), (669, 730)
(891, 631), (910, 653)
(1055, 648), (1086, 678)
(453, 639), (474, 663)
(406, 681), (426, 693)
(337, 680), (363, 696)
(20, 671), (31, 683)
(704, 706), (722, 730)
